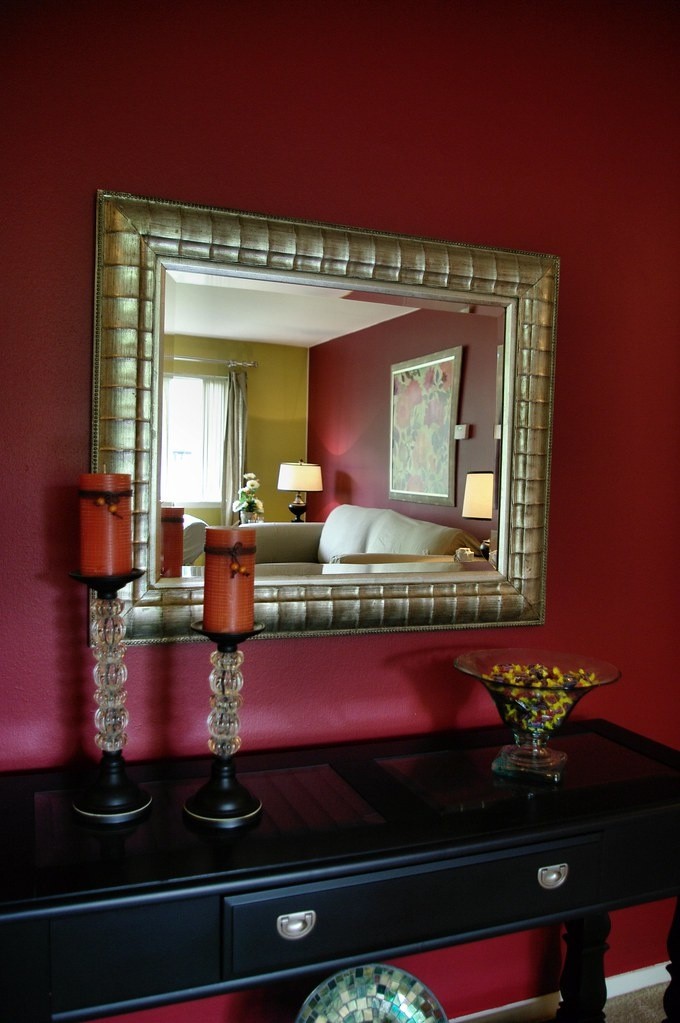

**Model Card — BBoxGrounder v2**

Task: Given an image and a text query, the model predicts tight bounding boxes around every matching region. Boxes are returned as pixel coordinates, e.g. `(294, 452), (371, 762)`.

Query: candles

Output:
(160, 497), (185, 579)
(203, 524), (256, 633)
(78, 459), (133, 577)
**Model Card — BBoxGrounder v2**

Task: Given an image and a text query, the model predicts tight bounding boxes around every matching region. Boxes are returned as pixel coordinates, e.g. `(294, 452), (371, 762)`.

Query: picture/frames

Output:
(388, 344), (463, 507)
(89, 189), (561, 646)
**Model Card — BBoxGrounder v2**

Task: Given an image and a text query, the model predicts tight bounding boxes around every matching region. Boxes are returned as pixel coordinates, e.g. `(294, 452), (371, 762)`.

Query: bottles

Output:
(247, 512), (264, 523)
(480, 540), (490, 562)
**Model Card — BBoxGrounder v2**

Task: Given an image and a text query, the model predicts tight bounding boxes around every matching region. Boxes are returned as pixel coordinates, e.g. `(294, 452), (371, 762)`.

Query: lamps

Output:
(276, 458), (323, 522)
(461, 470), (494, 562)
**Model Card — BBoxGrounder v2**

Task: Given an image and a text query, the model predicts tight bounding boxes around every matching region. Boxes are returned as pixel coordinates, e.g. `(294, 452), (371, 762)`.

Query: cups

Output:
(455, 548), (474, 561)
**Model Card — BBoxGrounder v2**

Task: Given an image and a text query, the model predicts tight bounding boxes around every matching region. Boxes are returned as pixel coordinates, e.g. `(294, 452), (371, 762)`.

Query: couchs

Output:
(237, 503), (483, 564)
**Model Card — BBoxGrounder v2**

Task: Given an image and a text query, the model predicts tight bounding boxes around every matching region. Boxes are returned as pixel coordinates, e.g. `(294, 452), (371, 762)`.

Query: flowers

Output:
(232, 472), (264, 514)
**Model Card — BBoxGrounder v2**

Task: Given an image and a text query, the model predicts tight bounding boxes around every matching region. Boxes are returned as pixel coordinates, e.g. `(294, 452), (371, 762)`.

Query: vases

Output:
(240, 510), (257, 525)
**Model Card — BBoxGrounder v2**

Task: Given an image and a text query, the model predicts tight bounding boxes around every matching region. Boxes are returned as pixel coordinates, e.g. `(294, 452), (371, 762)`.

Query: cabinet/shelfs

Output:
(1, 785), (679, 1023)
